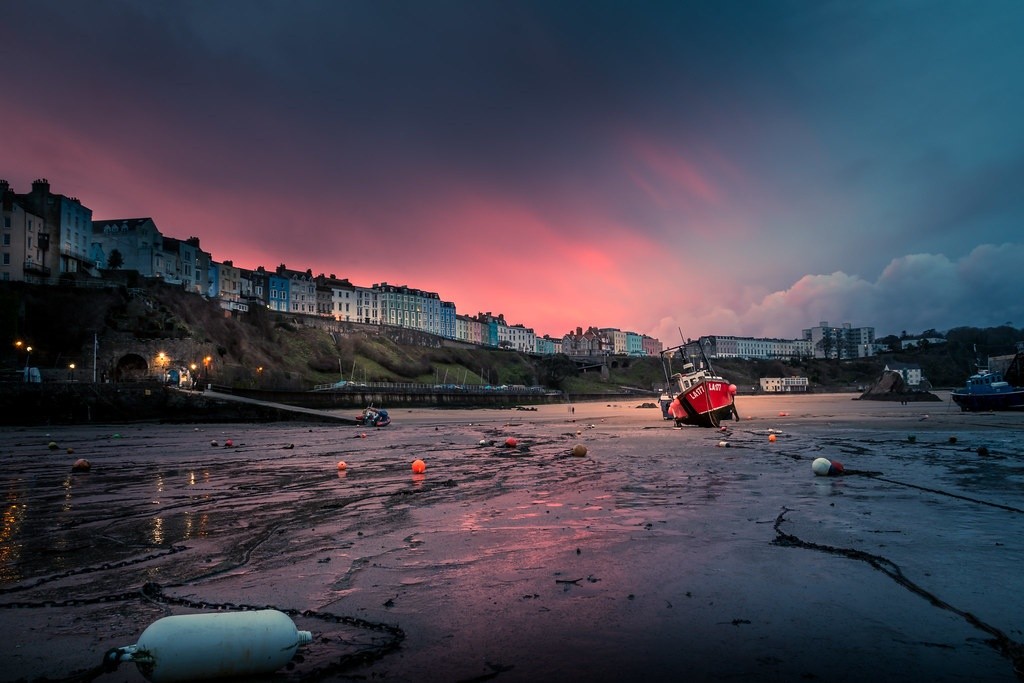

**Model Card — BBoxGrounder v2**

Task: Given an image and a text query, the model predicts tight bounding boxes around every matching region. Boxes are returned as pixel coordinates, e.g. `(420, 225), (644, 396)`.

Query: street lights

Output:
(191, 364), (196, 390)
(203, 355), (212, 389)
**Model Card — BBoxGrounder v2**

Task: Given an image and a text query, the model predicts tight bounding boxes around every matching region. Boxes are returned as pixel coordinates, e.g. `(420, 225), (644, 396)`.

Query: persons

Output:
(901, 389), (908, 405)
(190, 370), (199, 391)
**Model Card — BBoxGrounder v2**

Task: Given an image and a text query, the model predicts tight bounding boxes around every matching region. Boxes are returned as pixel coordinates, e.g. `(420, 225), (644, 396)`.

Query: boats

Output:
(355, 403), (391, 427)
(660, 393), (678, 421)
(950, 369), (1024, 412)
(659, 326), (734, 428)
